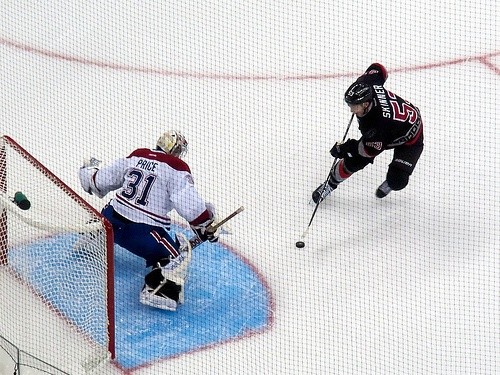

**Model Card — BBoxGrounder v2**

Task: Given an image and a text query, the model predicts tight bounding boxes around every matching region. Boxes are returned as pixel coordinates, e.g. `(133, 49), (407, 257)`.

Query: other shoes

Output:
(312, 181), (337, 204)
(69, 248), (96, 262)
(376, 181), (391, 198)
(140, 283), (177, 311)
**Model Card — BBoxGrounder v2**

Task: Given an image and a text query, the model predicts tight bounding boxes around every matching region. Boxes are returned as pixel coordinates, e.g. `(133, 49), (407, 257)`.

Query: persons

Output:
(312, 62), (424, 203)
(80, 129), (220, 310)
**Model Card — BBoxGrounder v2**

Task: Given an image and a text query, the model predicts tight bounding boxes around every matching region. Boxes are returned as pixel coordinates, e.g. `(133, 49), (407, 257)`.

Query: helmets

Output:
(345, 82), (372, 104)
(156, 130), (187, 155)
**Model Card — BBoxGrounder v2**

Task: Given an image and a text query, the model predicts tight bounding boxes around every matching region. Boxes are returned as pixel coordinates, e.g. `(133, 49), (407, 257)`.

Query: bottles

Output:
(15, 192), (30, 210)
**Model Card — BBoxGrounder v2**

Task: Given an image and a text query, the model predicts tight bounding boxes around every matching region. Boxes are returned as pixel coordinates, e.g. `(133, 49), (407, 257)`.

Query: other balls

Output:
(296, 241), (305, 248)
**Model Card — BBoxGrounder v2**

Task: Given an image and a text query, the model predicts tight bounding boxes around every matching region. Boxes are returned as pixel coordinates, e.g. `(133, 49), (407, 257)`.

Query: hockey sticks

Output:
(189, 206), (245, 250)
(301, 113), (355, 238)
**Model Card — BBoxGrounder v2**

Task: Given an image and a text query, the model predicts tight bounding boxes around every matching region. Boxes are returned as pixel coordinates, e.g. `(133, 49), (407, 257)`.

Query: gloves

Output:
(330, 138), (351, 159)
(194, 221), (221, 243)
